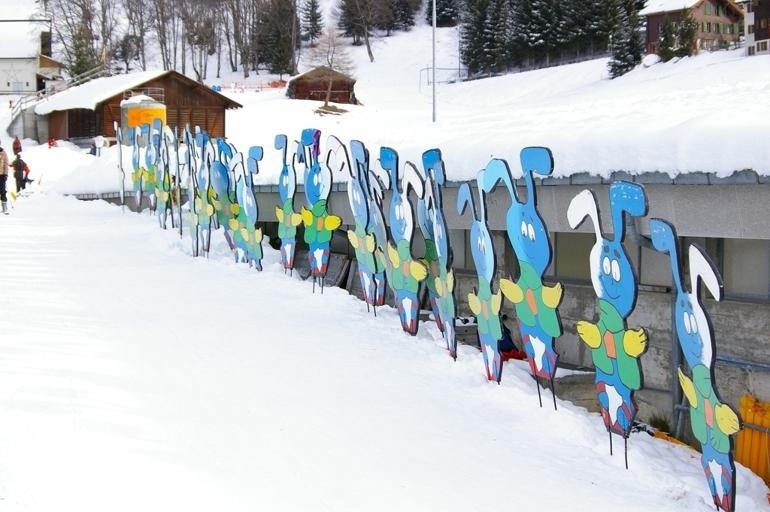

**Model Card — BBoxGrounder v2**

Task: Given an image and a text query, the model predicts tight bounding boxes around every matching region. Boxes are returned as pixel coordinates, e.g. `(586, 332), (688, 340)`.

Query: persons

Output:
(9, 155), (30, 193)
(0, 147), (8, 215)
(86, 144), (96, 155)
